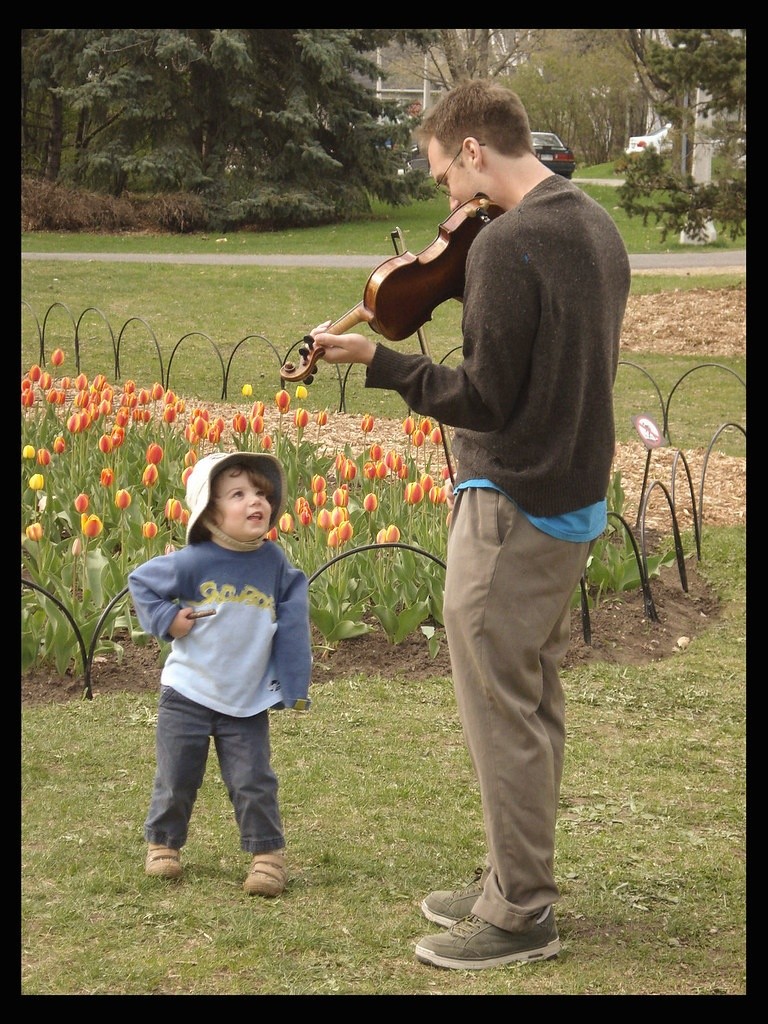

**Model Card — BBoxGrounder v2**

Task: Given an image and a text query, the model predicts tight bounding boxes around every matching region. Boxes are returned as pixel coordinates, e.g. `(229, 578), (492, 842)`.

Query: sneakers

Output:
(243, 853), (287, 895)
(422, 867), (485, 928)
(415, 905), (562, 971)
(146, 843), (182, 876)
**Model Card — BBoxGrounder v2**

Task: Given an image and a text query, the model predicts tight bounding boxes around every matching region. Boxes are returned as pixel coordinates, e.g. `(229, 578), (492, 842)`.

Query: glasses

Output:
(436, 144), (486, 197)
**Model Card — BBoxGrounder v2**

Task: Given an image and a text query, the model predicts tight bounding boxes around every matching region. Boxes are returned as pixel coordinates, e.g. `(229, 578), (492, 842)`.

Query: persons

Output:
(126, 451), (311, 893)
(307, 80), (631, 971)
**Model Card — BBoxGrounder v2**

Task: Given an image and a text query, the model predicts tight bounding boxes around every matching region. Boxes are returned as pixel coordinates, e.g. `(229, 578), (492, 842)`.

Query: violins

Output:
(280, 192), (506, 386)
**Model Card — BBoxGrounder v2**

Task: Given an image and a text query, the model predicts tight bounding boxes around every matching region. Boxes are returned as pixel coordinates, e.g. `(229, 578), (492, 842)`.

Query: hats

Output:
(184, 451), (287, 544)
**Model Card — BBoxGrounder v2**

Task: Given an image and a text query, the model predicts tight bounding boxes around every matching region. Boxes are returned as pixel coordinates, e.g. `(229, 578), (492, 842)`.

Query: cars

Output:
(530, 131), (576, 179)
(624, 122), (674, 160)
